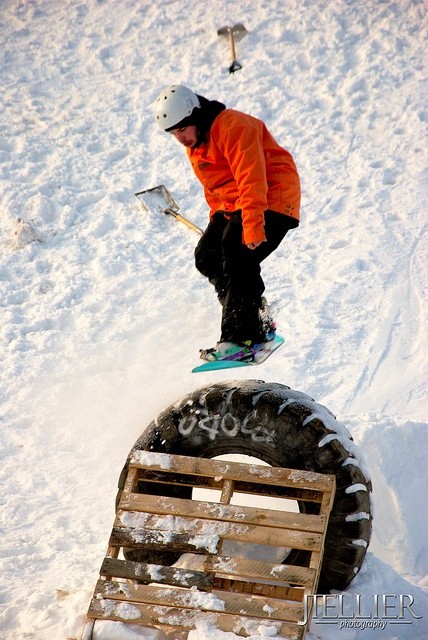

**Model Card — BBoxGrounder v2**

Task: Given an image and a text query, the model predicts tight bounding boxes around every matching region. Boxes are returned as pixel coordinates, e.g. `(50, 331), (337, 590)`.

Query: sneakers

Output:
(258, 297), (276, 342)
(200, 339), (254, 365)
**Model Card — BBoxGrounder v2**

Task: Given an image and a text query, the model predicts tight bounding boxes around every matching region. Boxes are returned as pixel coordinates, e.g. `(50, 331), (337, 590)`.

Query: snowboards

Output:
(192, 331), (285, 371)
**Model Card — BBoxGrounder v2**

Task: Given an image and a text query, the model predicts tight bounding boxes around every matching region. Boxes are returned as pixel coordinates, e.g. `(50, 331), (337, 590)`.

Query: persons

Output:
(154, 84), (302, 361)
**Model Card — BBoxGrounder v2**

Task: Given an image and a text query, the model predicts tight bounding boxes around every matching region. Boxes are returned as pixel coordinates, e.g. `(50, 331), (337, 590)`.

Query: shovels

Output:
(135, 185), (204, 236)
(218, 24), (247, 74)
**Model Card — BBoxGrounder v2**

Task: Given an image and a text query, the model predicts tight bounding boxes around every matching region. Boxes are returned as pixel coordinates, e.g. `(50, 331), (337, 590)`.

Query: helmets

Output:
(153, 85), (199, 131)
